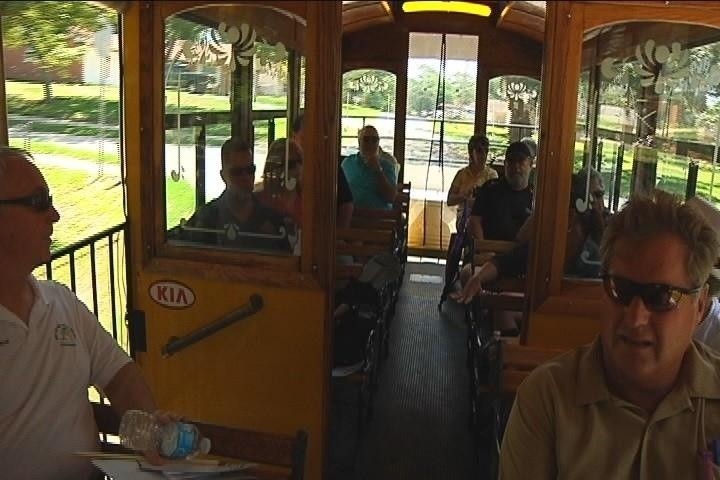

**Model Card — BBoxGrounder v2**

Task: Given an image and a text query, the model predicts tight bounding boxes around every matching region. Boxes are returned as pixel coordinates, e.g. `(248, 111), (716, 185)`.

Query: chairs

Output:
(449, 161), (604, 454)
(176, 181), (412, 469)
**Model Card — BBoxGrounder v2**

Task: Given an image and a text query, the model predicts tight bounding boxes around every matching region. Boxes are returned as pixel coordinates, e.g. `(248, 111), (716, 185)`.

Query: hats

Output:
(505, 140), (536, 158)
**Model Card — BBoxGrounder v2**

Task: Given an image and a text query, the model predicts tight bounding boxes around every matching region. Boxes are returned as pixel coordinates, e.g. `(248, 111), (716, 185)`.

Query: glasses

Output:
(270, 159), (299, 172)
(594, 190), (605, 200)
(229, 164), (257, 178)
(597, 269), (703, 311)
(0, 186), (54, 212)
(361, 135), (380, 144)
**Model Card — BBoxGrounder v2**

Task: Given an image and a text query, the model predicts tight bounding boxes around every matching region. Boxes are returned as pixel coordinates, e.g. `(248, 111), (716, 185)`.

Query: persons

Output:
(685, 198), (719, 353)
(0, 146), (191, 480)
(444, 132), (614, 336)
(498, 196), (720, 479)
(179, 113), (401, 251)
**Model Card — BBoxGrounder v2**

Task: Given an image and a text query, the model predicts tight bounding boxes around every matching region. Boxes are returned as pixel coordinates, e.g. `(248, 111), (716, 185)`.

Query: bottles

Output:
(119, 409), (211, 459)
